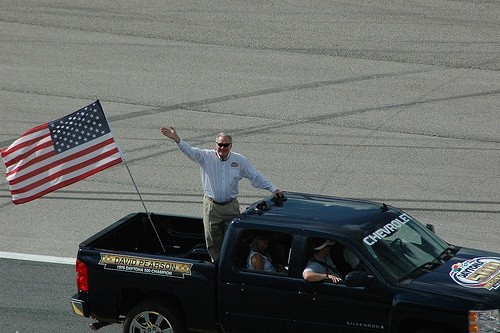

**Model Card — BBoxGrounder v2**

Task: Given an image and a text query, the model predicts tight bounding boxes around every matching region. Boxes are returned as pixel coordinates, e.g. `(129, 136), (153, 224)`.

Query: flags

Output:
(1, 97), (125, 206)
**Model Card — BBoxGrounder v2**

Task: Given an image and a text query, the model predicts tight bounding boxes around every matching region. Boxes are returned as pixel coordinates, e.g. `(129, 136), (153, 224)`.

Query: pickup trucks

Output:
(70, 190), (500, 333)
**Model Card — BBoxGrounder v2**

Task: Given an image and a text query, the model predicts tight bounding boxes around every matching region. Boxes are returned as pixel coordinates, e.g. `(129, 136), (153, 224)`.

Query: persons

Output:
(301, 237), (344, 283)
(245, 230), (287, 276)
(162, 119), (284, 274)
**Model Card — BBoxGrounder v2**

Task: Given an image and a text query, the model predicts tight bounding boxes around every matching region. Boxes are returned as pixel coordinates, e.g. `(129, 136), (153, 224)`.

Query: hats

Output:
(310, 238), (335, 250)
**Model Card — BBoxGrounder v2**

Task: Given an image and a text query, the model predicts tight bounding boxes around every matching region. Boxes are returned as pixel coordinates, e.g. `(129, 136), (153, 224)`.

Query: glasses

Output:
(216, 142), (231, 147)
